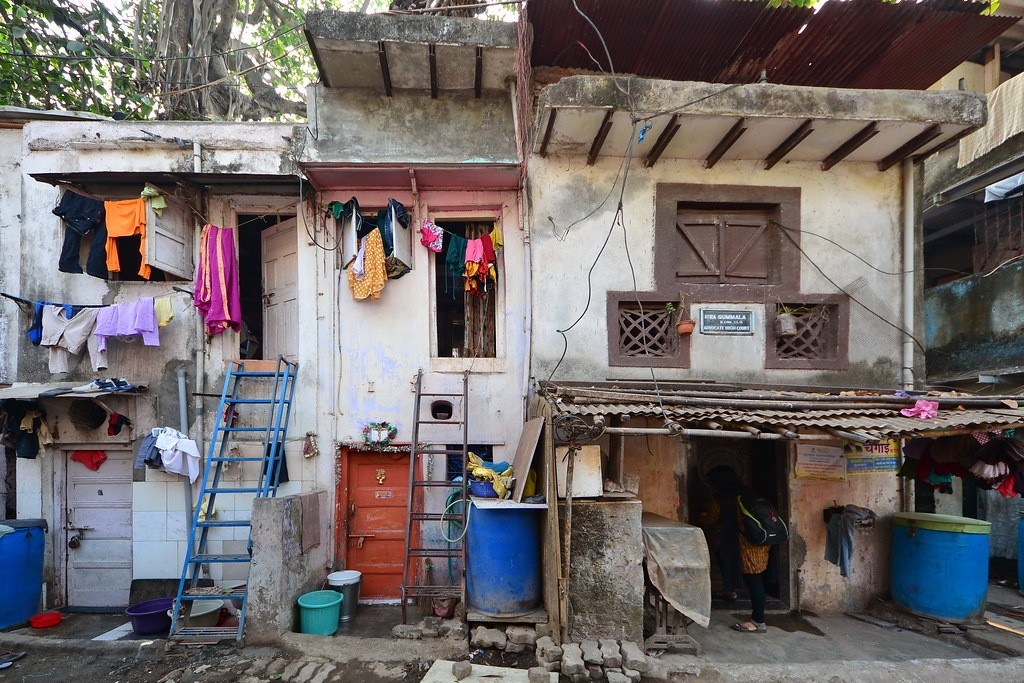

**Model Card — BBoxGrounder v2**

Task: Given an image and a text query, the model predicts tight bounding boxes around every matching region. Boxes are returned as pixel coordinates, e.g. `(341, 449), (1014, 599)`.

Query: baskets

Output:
(470, 480), (499, 498)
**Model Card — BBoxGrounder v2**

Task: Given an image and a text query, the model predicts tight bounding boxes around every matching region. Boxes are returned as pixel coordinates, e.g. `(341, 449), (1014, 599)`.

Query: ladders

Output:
(401, 370), (470, 626)
(166, 355), (299, 648)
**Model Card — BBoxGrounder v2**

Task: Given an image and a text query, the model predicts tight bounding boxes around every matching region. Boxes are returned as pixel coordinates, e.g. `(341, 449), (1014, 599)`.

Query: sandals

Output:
(732, 620), (766, 633)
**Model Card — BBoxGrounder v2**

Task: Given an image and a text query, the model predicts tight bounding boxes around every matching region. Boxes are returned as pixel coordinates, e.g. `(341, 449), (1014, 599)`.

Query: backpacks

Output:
(737, 495), (790, 546)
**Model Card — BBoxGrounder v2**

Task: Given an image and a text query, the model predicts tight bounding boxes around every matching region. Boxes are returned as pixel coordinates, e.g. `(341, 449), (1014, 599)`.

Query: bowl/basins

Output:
(167, 599), (224, 628)
(227, 583), (246, 610)
(29, 611), (62, 627)
(125, 597), (175, 636)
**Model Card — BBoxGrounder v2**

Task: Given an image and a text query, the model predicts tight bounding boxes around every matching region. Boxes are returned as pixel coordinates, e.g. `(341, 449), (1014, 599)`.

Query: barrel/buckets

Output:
(297, 589), (343, 636)
(464, 502), (544, 617)
(0, 517), (49, 629)
(1016, 510), (1024, 598)
(327, 569), (362, 622)
(889, 511), (993, 623)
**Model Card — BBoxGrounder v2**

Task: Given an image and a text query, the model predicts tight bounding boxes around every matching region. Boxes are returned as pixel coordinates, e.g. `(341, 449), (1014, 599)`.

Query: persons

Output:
(696, 464), (776, 632)
